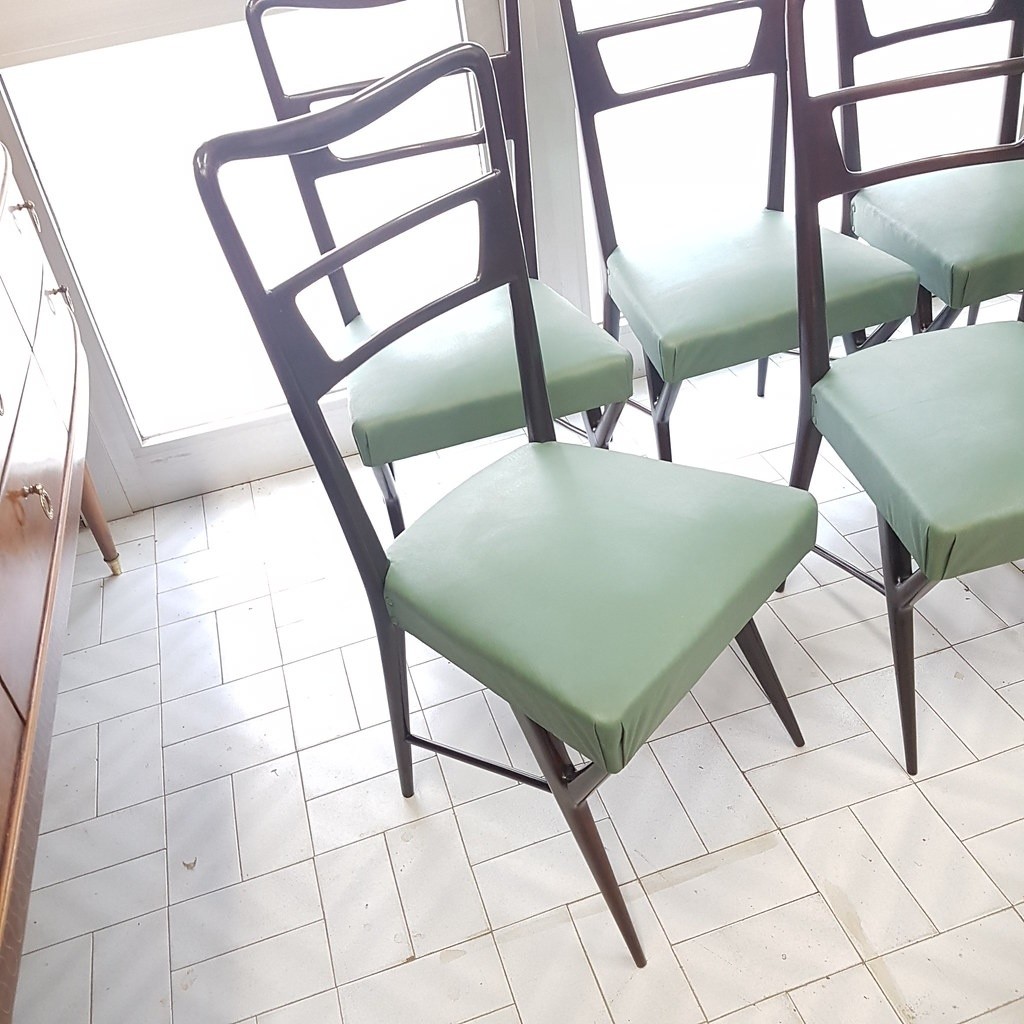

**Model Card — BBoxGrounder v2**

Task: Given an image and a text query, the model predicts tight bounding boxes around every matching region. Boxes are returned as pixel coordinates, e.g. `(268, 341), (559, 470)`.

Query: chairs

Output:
(192, 2), (1024, 966)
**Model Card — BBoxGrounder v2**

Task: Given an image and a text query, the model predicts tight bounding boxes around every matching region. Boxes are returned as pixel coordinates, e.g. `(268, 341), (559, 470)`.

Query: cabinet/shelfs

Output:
(0, 138), (123, 1024)
